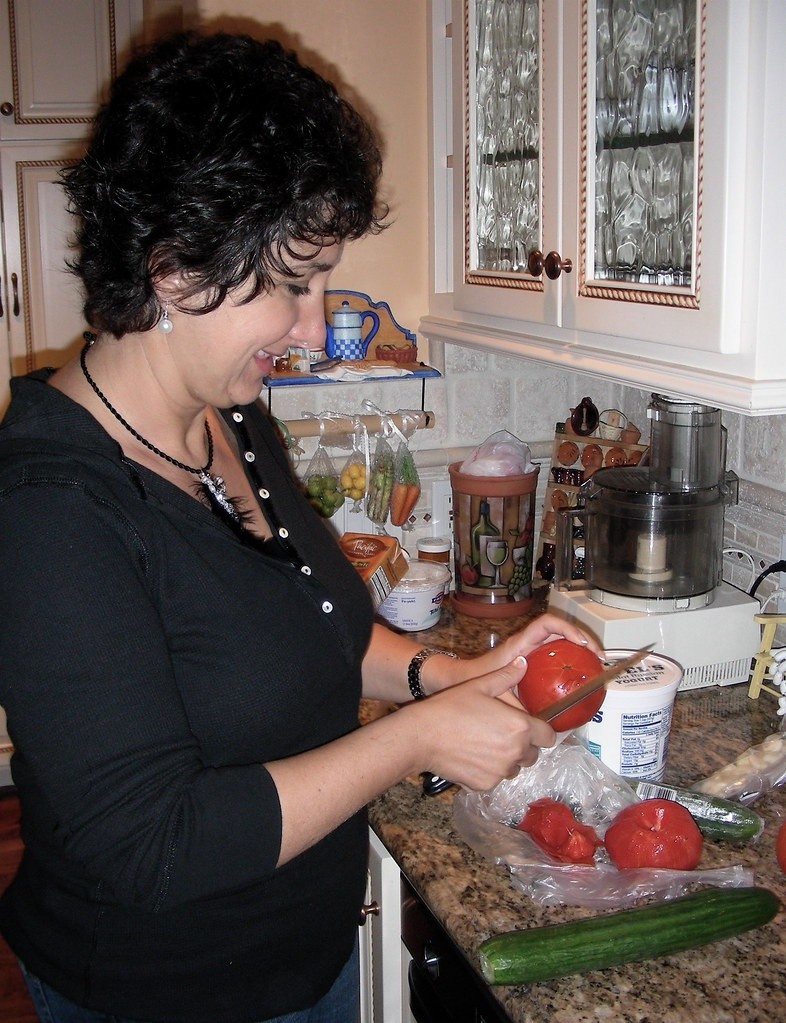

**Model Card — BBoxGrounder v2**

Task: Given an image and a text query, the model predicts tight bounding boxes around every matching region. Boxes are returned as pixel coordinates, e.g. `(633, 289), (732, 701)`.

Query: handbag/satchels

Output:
(452, 729), (755, 904)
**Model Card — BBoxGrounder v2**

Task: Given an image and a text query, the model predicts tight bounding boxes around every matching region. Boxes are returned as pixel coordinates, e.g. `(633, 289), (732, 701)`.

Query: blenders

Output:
(547, 392), (761, 693)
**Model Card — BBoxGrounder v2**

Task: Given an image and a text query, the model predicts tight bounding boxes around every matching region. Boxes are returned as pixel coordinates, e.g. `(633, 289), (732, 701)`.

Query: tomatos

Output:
(514, 639), (609, 734)
(603, 797), (704, 872)
(776, 816), (786, 877)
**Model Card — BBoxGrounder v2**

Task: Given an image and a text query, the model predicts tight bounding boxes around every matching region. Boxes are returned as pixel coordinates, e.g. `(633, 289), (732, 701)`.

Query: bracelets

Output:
(407, 646), (455, 699)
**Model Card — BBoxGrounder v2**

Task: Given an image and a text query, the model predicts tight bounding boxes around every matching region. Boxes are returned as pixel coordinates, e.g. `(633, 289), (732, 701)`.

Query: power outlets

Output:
(776, 534), (786, 628)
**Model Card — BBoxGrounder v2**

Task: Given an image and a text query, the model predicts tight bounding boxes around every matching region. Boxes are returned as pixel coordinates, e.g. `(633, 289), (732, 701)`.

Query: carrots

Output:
(390, 479), (421, 526)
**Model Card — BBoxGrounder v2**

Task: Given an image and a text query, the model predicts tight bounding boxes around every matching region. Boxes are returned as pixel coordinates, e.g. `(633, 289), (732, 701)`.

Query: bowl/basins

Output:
(376, 559), (452, 631)
(571, 652), (683, 781)
(309, 348), (324, 364)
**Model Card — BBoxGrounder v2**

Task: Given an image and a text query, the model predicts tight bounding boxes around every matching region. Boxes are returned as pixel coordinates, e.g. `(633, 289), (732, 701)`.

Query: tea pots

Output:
(325, 300), (380, 362)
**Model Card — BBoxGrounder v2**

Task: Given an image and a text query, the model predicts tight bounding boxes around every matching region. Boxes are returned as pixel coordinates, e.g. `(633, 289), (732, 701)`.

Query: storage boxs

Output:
(339, 531), (409, 616)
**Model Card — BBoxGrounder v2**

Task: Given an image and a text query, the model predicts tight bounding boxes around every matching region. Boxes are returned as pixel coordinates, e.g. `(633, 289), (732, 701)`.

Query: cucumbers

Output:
(616, 774), (765, 841)
(479, 885), (781, 986)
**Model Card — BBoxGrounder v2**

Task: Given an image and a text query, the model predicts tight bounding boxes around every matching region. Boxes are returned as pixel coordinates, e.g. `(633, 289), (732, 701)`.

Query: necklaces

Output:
(80, 331), (255, 534)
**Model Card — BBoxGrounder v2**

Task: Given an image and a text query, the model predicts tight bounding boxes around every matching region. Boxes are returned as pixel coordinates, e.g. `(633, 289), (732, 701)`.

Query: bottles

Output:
(416, 537), (451, 597)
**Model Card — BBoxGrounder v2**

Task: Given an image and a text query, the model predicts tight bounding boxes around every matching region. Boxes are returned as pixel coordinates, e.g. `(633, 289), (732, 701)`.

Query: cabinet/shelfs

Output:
(0, 0), (147, 426)
(357, 830), (523, 1023)
(416, 0), (786, 416)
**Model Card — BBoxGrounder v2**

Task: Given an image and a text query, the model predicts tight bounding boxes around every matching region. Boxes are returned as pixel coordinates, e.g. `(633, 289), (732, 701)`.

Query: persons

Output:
(0, 14), (608, 1023)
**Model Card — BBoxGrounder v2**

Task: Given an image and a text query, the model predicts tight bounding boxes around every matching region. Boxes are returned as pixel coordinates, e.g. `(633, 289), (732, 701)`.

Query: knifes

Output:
(422, 650), (656, 797)
